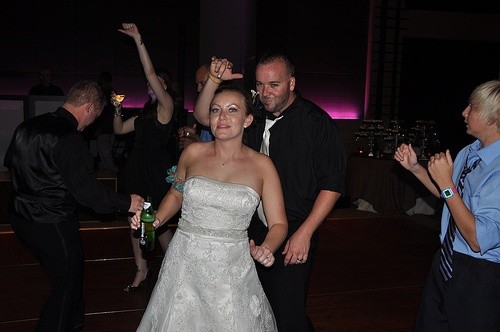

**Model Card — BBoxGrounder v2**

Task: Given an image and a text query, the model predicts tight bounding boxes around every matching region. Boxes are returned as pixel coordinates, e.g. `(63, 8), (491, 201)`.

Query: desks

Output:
(350, 153), (417, 215)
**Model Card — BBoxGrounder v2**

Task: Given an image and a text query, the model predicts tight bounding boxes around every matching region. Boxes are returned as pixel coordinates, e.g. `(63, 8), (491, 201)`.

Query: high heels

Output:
(124, 268), (152, 294)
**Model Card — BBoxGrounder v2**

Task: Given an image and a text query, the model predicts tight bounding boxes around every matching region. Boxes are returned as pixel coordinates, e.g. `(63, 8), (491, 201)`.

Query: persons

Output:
(193, 53), (346, 332)
(135, 86), (288, 332)
(3, 82), (147, 332)
(110, 21), (184, 294)
(178, 64), (260, 152)
(393, 79), (500, 332)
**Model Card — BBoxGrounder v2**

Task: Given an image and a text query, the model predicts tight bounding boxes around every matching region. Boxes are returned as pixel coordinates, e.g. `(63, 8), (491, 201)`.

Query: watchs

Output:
(440, 188), (457, 199)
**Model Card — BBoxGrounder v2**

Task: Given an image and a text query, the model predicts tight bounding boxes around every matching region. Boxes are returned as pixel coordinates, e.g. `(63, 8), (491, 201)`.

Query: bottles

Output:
(139, 194), (156, 252)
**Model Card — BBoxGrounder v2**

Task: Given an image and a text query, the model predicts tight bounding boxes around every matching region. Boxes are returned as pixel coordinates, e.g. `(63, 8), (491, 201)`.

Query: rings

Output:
(297, 258), (303, 261)
(267, 257), (271, 262)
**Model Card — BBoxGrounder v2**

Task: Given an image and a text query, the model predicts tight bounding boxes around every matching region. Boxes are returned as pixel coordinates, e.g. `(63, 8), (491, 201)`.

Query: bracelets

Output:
(114, 114), (124, 117)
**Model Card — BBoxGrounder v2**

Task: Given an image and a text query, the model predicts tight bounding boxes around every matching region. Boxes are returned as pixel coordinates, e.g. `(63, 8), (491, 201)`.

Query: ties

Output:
(256, 115), (285, 227)
(437, 155), (482, 282)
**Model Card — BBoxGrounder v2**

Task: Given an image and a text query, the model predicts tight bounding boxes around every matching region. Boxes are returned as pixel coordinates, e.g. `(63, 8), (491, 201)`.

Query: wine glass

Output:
(113, 95), (126, 117)
